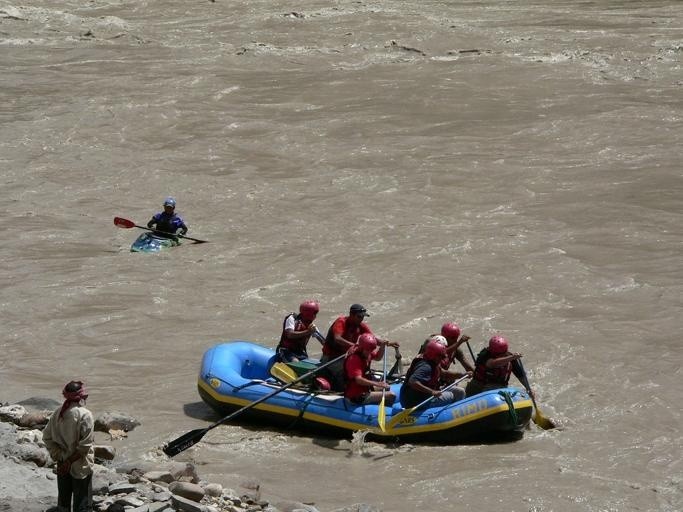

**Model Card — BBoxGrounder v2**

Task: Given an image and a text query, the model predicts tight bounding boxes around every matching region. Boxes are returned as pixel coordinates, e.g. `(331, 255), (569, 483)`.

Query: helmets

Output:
(489, 335), (509, 358)
(162, 197), (176, 208)
(424, 340), (447, 363)
(432, 335), (449, 346)
(441, 322), (461, 340)
(299, 301), (320, 321)
(359, 334), (378, 354)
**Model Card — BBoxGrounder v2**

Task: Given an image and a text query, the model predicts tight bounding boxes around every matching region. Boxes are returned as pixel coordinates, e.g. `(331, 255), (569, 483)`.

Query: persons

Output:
(42, 380), (95, 511)
(147, 197), (188, 238)
(319, 304), (400, 388)
(276, 301), (325, 363)
(343, 333), (396, 407)
(418, 323), (474, 372)
(399, 340), (474, 411)
(465, 336), (535, 399)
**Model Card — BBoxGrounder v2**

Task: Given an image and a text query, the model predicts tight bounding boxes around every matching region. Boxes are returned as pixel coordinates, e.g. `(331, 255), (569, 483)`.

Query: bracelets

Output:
(65, 456), (75, 465)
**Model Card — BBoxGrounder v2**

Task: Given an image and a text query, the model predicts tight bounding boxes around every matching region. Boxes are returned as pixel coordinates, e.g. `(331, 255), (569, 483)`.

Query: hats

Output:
(350, 304), (370, 318)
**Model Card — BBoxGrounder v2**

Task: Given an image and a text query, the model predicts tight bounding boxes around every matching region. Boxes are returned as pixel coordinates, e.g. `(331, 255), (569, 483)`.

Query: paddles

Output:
(163, 346), (353, 456)
(378, 343), (387, 432)
(386, 374), (470, 429)
(115, 217), (204, 244)
(518, 356), (555, 430)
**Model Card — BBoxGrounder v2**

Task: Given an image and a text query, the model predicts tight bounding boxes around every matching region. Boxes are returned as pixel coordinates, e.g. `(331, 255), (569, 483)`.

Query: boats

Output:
(197, 341), (532, 431)
(129, 228), (183, 255)
